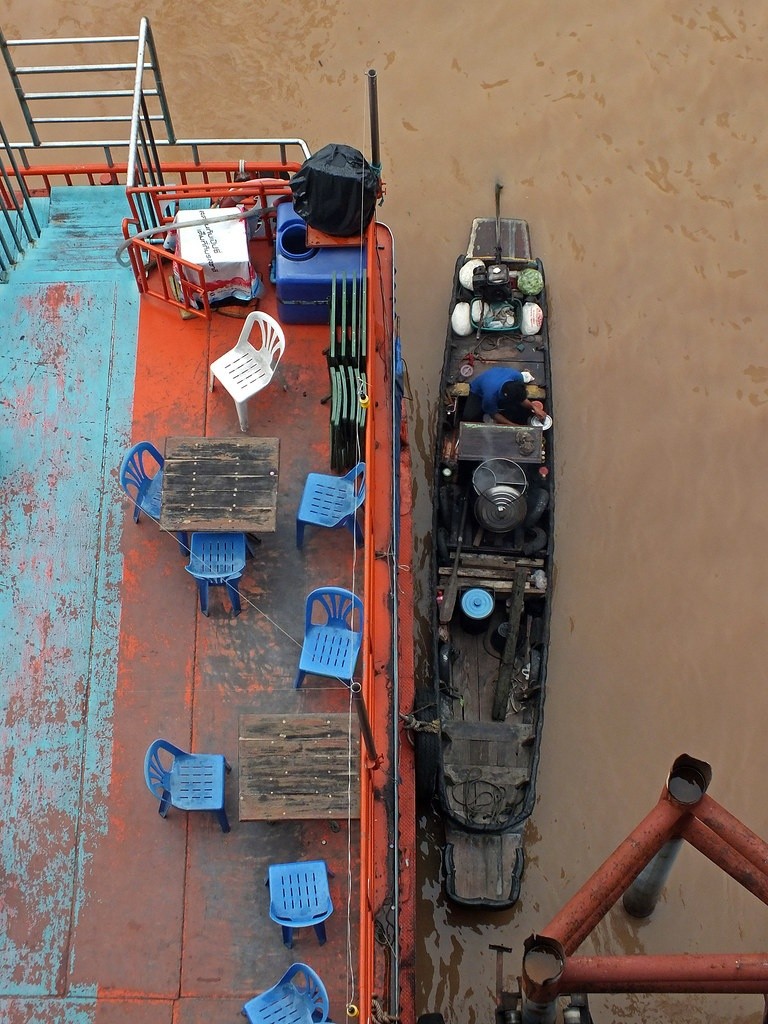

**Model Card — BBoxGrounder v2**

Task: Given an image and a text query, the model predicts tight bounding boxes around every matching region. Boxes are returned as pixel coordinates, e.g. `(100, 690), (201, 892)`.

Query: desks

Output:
(238, 712), (361, 832)
(158, 435), (281, 533)
(456, 422), (543, 483)
(320, 268), (367, 403)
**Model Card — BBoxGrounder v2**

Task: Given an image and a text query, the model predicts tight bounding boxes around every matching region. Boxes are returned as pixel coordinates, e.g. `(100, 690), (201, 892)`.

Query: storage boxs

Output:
(163, 203), (264, 311)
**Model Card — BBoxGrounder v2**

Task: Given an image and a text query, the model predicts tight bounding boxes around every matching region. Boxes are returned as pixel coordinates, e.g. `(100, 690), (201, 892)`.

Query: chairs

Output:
(296, 462), (366, 548)
(263, 860), (335, 950)
(144, 739), (232, 834)
(208, 310), (286, 432)
(294, 587), (364, 700)
(119, 441), (191, 556)
(185, 531), (256, 618)
(241, 963), (332, 1024)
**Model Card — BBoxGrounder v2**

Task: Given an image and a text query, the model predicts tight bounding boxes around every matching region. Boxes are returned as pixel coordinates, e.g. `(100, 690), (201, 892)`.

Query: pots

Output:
(531, 414), (552, 430)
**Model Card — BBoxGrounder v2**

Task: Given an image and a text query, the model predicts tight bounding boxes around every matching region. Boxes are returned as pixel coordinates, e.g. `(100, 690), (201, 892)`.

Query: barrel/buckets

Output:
(458, 584), (495, 634)
(472, 457), (528, 533)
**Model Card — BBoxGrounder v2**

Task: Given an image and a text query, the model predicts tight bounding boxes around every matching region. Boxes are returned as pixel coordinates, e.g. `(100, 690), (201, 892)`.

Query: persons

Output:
(463, 367), (547, 427)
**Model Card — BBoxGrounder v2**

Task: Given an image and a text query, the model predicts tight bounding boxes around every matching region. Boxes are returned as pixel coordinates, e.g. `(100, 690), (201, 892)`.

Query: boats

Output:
(417, 213), (555, 907)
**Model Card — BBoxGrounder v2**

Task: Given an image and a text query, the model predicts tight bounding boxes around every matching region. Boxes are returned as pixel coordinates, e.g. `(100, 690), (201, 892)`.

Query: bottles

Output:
(437, 592), (443, 612)
